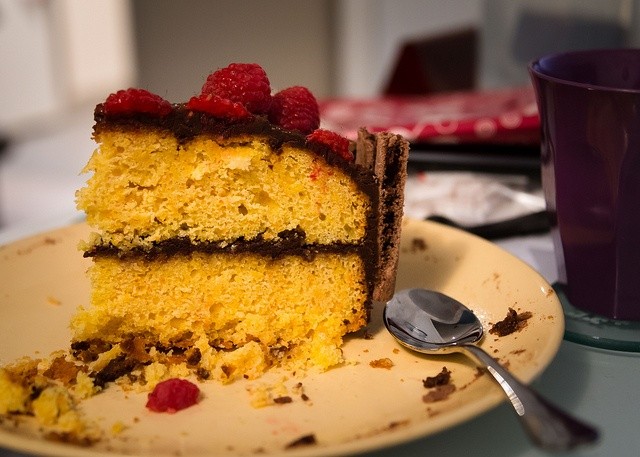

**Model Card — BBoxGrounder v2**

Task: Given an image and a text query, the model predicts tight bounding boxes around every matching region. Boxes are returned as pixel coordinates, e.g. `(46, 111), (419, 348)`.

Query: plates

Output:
(1, 215), (565, 456)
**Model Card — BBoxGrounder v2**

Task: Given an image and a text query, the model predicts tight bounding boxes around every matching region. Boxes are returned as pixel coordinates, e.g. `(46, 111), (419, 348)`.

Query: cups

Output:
(529, 49), (640, 325)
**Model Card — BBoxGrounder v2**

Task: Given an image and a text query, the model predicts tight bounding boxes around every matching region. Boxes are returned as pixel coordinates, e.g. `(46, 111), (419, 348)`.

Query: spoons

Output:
(383, 288), (601, 453)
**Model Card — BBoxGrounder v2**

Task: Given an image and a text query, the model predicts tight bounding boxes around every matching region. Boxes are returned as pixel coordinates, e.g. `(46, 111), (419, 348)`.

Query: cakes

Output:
(70, 63), (409, 379)
